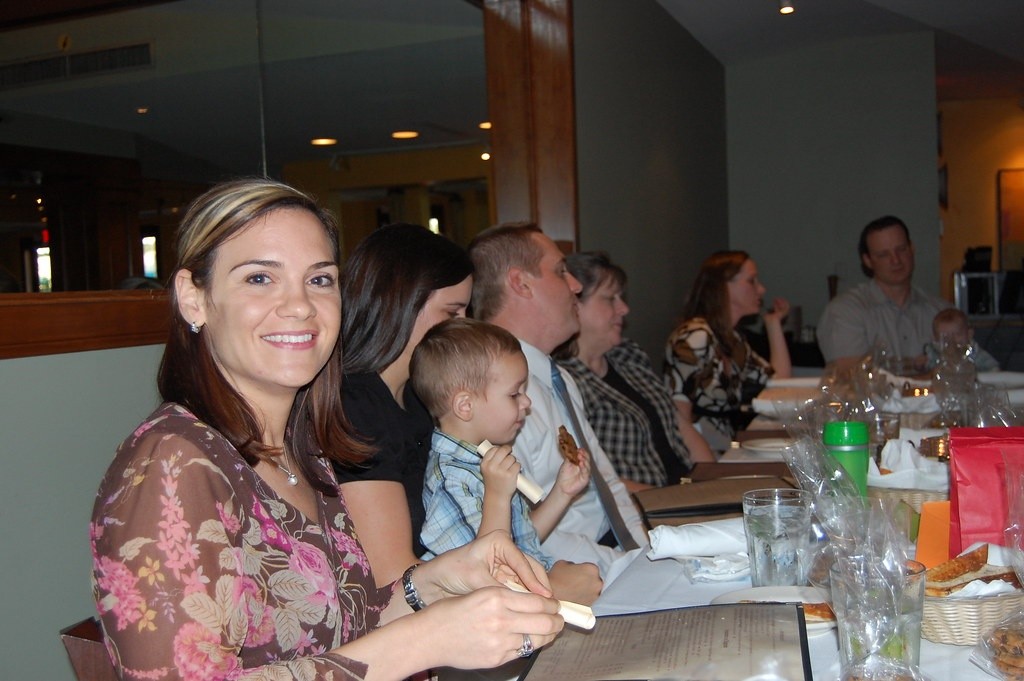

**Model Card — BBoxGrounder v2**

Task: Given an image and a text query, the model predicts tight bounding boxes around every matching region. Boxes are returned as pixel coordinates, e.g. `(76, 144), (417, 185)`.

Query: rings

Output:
(516, 634), (534, 657)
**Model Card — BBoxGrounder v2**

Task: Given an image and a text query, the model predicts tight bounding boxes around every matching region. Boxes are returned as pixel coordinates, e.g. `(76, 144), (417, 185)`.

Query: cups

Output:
(742, 489), (811, 585)
(828, 555), (926, 674)
(867, 411), (900, 455)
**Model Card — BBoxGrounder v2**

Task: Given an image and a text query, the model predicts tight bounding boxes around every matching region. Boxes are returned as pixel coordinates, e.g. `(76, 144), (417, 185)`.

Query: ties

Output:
(549, 357), (641, 552)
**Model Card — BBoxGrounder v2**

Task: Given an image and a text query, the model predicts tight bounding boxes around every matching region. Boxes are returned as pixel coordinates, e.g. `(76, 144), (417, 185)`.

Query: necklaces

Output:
(262, 443), (298, 486)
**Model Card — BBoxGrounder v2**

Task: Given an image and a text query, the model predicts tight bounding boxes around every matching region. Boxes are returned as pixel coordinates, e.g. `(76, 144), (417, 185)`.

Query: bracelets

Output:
(402, 563), (429, 612)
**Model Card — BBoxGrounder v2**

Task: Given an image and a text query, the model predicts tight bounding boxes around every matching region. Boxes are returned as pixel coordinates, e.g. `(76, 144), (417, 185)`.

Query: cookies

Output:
(558, 425), (580, 465)
(984, 626), (1024, 681)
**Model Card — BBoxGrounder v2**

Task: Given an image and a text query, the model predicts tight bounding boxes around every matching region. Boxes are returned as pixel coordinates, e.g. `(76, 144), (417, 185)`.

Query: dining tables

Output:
(517, 414), (1024, 681)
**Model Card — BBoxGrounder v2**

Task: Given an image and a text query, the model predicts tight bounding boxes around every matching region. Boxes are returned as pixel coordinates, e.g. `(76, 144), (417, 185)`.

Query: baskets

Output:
(920, 591), (1024, 647)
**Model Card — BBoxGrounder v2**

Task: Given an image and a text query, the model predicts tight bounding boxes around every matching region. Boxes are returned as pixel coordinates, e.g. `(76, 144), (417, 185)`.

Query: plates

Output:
(742, 438), (792, 451)
(709, 585), (837, 639)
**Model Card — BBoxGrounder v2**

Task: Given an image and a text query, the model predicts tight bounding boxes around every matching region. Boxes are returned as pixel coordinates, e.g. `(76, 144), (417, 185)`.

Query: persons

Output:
(89, 179), (714, 681)
(817, 216), (1001, 374)
(661, 249), (792, 454)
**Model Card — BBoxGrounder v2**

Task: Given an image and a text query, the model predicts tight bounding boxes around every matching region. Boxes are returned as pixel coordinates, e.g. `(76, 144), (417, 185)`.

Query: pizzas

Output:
(803, 602), (835, 619)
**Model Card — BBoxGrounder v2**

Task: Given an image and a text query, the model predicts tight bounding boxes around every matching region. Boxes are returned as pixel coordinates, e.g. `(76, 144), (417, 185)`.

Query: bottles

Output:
(824, 421), (869, 499)
(902, 382), (912, 397)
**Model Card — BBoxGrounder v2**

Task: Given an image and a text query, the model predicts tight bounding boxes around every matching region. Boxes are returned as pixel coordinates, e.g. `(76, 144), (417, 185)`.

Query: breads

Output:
(907, 543), (1023, 596)
